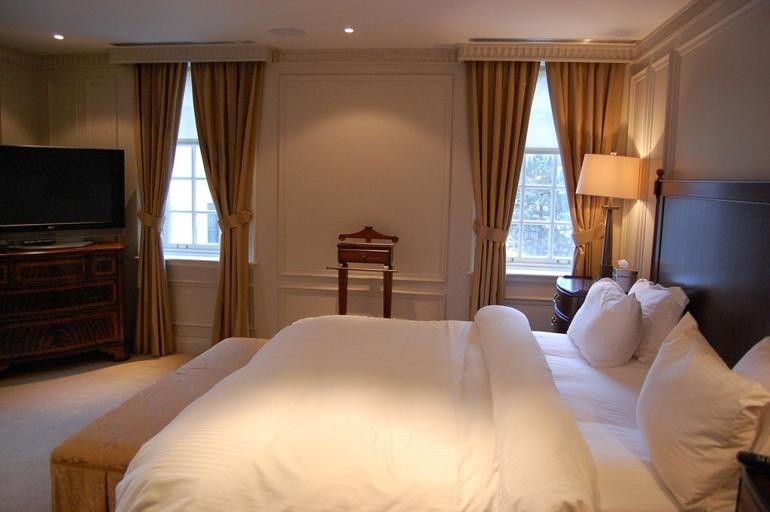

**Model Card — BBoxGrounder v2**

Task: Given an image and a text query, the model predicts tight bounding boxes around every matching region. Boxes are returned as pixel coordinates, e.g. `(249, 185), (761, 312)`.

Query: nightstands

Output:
(550, 276), (599, 332)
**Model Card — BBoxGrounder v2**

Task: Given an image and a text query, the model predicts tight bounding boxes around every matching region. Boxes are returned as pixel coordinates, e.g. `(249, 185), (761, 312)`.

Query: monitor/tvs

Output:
(0, 144), (126, 249)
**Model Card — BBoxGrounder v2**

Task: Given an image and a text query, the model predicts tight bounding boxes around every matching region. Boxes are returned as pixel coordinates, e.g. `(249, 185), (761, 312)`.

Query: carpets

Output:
(1, 353), (194, 512)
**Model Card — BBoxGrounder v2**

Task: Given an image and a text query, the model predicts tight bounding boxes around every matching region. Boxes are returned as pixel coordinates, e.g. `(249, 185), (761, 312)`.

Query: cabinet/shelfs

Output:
(0, 242), (130, 381)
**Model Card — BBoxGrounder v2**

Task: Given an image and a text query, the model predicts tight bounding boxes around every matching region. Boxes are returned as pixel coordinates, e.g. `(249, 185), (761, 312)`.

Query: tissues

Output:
(613, 260), (638, 293)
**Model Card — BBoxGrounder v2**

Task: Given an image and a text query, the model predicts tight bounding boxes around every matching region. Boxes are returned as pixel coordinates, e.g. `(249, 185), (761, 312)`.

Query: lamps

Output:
(572, 152), (641, 281)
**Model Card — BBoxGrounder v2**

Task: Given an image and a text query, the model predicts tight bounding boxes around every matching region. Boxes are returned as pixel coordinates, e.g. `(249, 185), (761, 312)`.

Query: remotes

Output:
(22, 239), (56, 245)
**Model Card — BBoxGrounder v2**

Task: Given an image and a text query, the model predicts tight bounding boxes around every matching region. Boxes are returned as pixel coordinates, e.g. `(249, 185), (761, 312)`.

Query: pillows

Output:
(625, 278), (692, 366)
(567, 278), (645, 372)
(629, 311), (767, 509)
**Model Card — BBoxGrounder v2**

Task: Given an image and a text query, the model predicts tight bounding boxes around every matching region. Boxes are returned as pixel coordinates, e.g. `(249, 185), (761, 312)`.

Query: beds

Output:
(53, 169), (768, 512)
